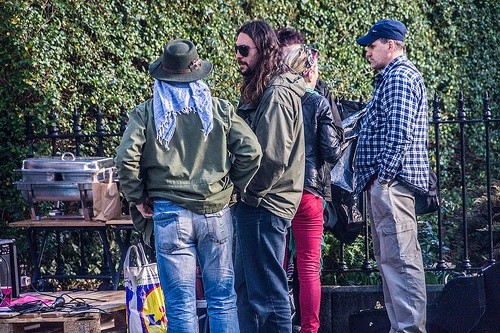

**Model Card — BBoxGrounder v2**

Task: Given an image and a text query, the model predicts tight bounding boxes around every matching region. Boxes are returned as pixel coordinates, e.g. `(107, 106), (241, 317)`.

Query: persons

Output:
(113, 40), (264, 333)
(229, 20), (306, 333)
(279, 40), (344, 333)
(342, 19), (431, 333)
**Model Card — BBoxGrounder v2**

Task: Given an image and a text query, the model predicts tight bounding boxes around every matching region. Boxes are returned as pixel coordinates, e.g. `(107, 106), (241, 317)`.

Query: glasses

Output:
(235, 45), (257, 57)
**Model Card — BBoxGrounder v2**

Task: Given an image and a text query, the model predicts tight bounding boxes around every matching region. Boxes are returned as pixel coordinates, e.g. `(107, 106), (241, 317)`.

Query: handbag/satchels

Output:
(92, 168), (120, 221)
(123, 243), (168, 333)
(413, 172), (439, 214)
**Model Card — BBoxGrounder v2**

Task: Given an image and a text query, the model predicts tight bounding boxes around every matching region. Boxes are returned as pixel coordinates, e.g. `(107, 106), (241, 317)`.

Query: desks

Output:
(8, 215), (133, 291)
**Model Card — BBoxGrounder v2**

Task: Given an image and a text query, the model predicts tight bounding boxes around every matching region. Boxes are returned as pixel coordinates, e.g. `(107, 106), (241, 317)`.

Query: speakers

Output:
(0, 239), (19, 301)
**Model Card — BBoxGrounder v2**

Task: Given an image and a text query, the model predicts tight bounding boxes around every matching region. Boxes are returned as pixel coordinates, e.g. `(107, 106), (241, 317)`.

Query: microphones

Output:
(46, 297), (65, 311)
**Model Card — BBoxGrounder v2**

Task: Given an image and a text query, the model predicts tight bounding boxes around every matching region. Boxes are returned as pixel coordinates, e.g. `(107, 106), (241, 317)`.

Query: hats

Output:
(149, 40), (213, 83)
(356, 19), (407, 45)
